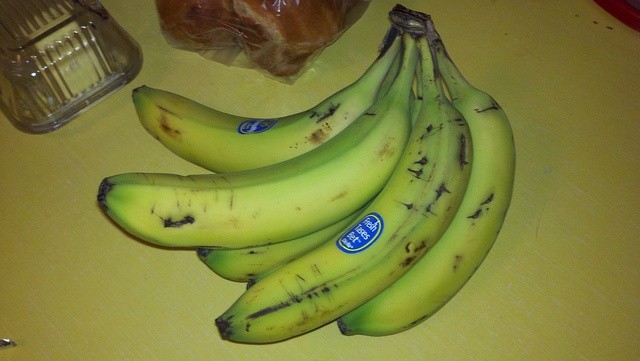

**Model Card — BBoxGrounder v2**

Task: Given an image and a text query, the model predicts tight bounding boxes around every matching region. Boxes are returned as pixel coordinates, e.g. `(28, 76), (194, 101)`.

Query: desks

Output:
(0, 1), (639, 361)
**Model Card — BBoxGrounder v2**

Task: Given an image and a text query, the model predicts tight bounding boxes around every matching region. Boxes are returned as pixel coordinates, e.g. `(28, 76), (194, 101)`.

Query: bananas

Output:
(97, 5), (516, 345)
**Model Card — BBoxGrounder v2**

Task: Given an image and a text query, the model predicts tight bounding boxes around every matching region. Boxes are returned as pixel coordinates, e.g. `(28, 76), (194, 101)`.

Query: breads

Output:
(148, 2), (371, 83)
